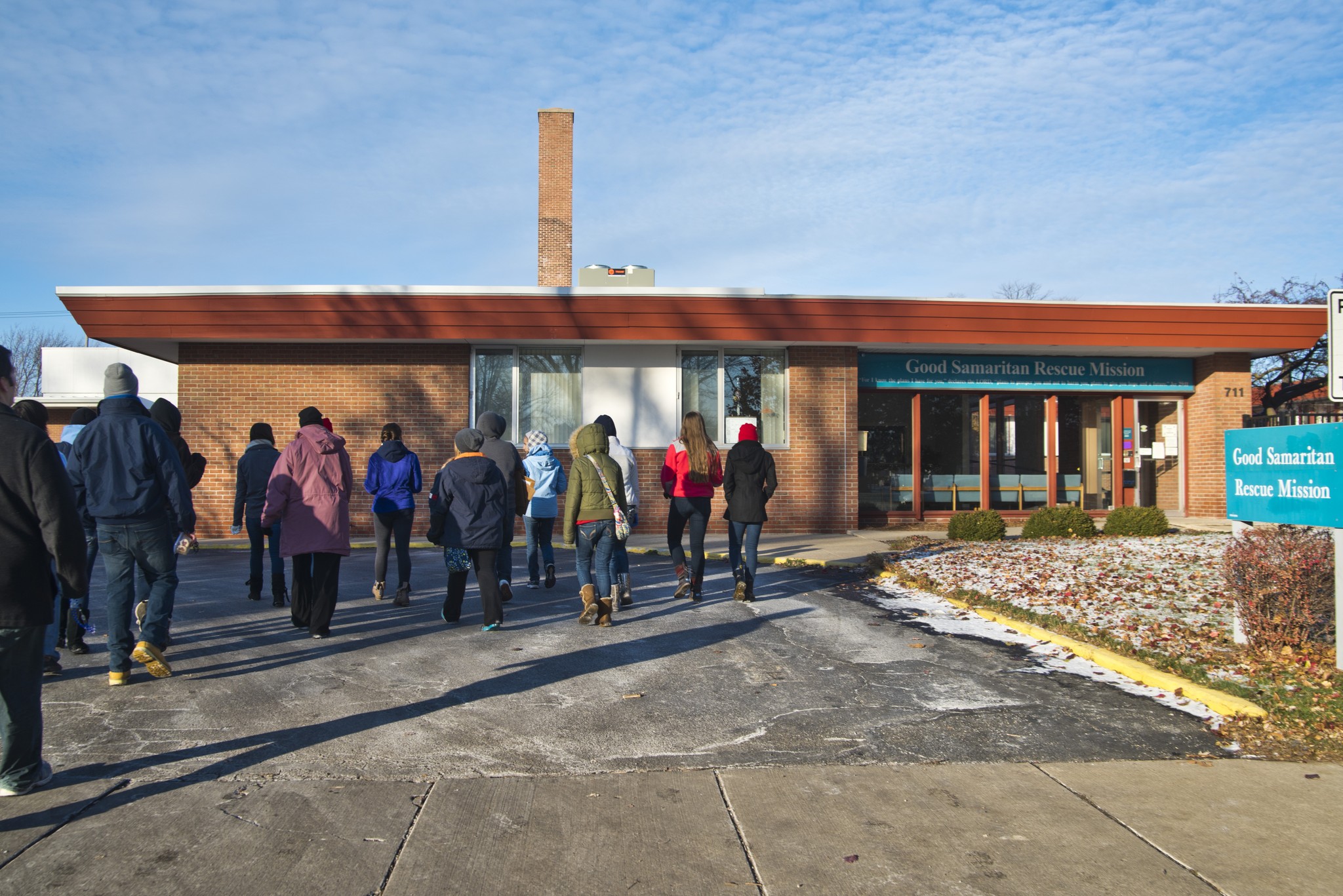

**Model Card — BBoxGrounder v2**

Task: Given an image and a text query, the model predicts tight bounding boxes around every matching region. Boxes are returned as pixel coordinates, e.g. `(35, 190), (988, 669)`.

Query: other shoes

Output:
(109, 600), (173, 687)
(0, 759), (54, 797)
(441, 563), (557, 631)
(43, 656), (62, 672)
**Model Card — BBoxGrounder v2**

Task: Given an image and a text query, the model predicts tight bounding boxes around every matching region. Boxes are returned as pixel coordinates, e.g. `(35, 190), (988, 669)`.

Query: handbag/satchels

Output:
(612, 503), (632, 541)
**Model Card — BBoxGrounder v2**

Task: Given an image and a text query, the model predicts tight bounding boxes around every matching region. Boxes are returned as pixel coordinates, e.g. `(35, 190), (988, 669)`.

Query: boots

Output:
(66, 608), (90, 653)
(392, 581), (412, 606)
(271, 573), (290, 606)
(578, 561), (756, 628)
(245, 572), (263, 601)
(56, 597), (68, 648)
(372, 579), (386, 600)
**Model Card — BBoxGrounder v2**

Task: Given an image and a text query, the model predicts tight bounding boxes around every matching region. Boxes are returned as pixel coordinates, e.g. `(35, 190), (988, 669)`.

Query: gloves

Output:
(231, 525), (243, 535)
(631, 513), (639, 528)
(663, 491), (673, 499)
(261, 527), (273, 537)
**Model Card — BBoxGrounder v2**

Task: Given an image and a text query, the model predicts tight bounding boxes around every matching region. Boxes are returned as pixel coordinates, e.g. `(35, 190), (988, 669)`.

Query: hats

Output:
(322, 418), (333, 433)
(455, 428), (485, 453)
(298, 406), (323, 429)
(593, 415), (616, 437)
(103, 362), (139, 399)
(738, 423), (758, 442)
(69, 407), (97, 425)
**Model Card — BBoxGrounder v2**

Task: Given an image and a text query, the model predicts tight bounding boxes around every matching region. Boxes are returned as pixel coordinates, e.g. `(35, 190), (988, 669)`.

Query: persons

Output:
(474, 411), (528, 603)
(135, 398), (207, 634)
(260, 407), (352, 639)
(363, 423), (422, 606)
(521, 430), (567, 589)
(64, 363), (197, 688)
(427, 428), (505, 632)
(10, 399), (64, 678)
(47, 407), (100, 654)
(723, 422), (778, 601)
(232, 422), (291, 607)
(661, 411), (724, 602)
(563, 424), (627, 628)
(591, 415), (640, 613)
(0, 345), (87, 796)
